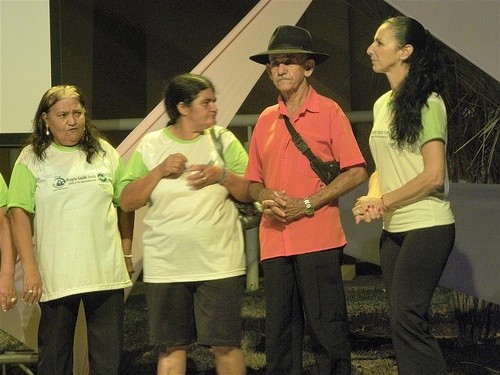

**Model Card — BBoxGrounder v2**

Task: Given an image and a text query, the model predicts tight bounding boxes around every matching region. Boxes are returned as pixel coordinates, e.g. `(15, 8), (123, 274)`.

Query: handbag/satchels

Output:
(234, 198), (261, 228)
(318, 162), (345, 186)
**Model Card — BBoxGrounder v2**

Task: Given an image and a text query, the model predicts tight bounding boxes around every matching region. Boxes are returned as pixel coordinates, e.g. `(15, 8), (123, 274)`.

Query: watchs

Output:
(303, 197), (314, 217)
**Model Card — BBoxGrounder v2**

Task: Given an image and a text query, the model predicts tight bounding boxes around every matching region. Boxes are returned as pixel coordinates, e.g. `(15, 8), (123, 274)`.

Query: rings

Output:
(202, 177), (206, 184)
(29, 290), (33, 293)
(11, 297), (15, 303)
(200, 171), (204, 176)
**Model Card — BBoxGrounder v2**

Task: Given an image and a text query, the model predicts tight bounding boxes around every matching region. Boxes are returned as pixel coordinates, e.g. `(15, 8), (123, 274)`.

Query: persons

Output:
(6, 84), (136, 375)
(0, 172), (18, 312)
(353, 16), (461, 375)
(114, 75), (258, 375)
(243, 26), (370, 375)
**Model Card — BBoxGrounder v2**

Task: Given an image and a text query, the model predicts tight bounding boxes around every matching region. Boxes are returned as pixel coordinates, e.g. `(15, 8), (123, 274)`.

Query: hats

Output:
(249, 25), (329, 66)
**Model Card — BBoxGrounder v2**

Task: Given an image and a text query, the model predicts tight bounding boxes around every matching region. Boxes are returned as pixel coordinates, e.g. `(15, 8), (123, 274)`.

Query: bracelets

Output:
(124, 255), (132, 258)
(219, 167), (228, 183)
(381, 195), (390, 211)
